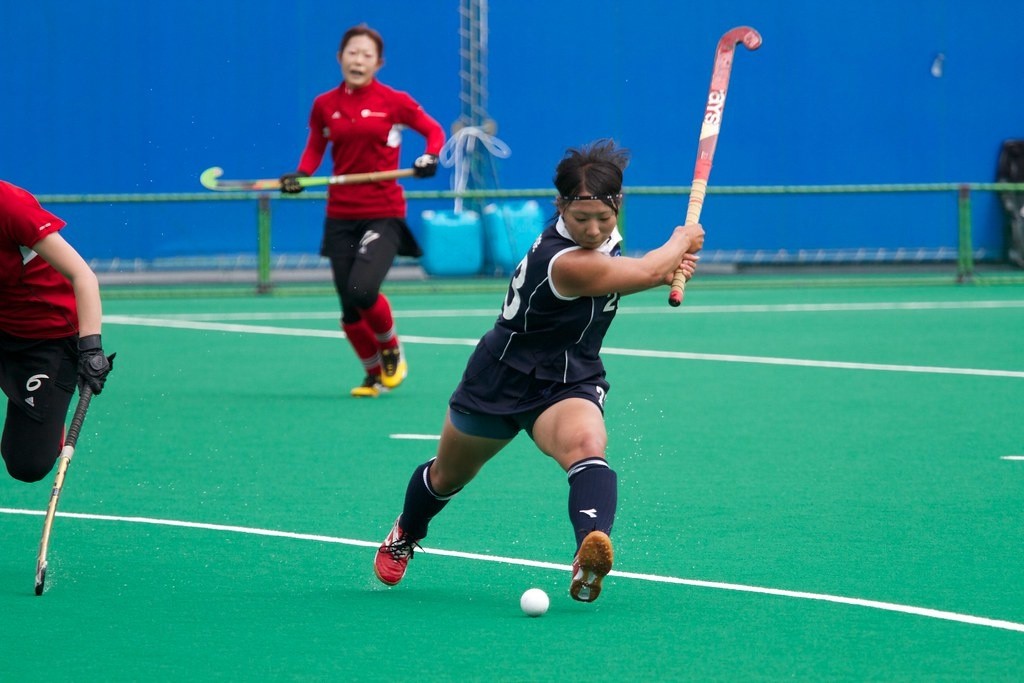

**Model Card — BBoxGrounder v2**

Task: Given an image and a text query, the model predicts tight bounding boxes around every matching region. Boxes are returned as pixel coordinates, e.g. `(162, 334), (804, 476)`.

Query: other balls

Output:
(521, 587), (551, 619)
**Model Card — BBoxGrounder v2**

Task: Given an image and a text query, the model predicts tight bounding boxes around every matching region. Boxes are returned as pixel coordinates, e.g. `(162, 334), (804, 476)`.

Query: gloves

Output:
(280, 170), (310, 193)
(413, 152), (439, 178)
(77, 332), (111, 396)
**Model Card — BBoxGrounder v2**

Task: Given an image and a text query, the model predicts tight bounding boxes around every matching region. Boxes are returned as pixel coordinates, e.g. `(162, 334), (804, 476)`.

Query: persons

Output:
(0, 178), (109, 483)
(373, 152), (704, 603)
(277, 27), (445, 398)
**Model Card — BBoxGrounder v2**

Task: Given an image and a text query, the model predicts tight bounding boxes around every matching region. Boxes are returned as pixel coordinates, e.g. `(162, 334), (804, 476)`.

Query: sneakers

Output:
(569, 530), (614, 603)
(349, 371), (388, 398)
(372, 508), (427, 586)
(379, 344), (407, 387)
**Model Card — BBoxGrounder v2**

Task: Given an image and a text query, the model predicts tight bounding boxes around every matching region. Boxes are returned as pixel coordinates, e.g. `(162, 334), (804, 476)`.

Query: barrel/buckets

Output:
(483, 201), (543, 271)
(420, 209), (483, 275)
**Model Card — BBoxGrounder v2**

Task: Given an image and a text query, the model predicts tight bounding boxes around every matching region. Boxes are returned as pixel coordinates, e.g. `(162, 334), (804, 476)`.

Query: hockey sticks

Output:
(199, 164), (417, 193)
(32, 382), (90, 597)
(665, 25), (761, 307)
